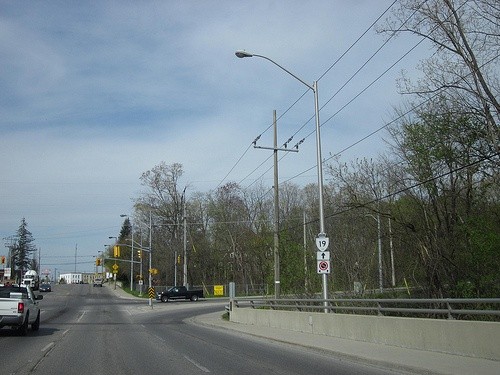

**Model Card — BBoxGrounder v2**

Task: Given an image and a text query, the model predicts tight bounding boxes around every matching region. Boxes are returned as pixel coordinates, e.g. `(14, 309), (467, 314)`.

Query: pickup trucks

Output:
(0, 283), (43, 336)
(156, 285), (205, 303)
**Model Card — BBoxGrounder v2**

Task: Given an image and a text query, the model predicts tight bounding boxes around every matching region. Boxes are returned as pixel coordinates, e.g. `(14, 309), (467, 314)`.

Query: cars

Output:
(39, 284), (51, 292)
(94, 278), (102, 287)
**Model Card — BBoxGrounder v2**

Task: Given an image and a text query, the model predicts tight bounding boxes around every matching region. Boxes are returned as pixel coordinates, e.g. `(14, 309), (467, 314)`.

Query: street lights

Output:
(92, 223), (142, 291)
(236, 51), (329, 313)
(120, 210), (151, 288)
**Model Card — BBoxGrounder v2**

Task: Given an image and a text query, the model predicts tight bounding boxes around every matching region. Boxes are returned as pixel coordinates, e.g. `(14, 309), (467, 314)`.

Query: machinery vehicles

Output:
(20, 270), (39, 290)
